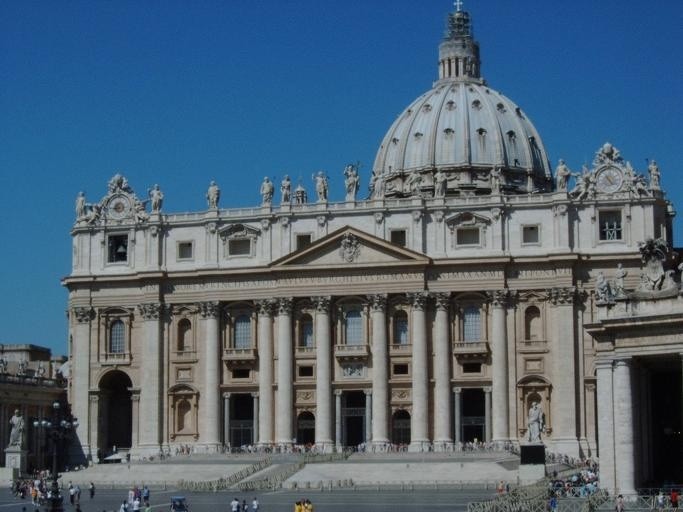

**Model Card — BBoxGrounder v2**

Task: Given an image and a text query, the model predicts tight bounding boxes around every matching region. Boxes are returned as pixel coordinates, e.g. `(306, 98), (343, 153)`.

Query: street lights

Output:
(33, 400), (78, 511)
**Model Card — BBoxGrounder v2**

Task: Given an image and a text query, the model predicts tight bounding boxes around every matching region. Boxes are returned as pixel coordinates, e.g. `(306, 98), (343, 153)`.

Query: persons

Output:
(149, 183), (164, 212)
(432, 171), (456, 198)
(402, 167), (422, 194)
(311, 169), (329, 202)
(372, 168), (387, 198)
(486, 169), (503, 195)
(279, 174), (291, 203)
(614, 495), (625, 512)
(300, 498), (306, 512)
(625, 166), (653, 200)
(294, 501), (301, 512)
(170, 502), (177, 508)
(8, 467), (83, 512)
(206, 181), (220, 209)
(240, 499), (249, 512)
(177, 501), (185, 509)
(646, 158), (661, 186)
(342, 164), (359, 192)
(119, 483), (153, 512)
(73, 190), (85, 214)
(8, 409), (25, 447)
(669, 489), (681, 512)
(229, 498), (242, 512)
(88, 481), (95, 499)
(567, 163), (595, 201)
(526, 401), (544, 443)
(250, 497), (260, 512)
(593, 261), (628, 302)
(546, 459), (599, 512)
(497, 480), (504, 494)
(552, 158), (570, 191)
(258, 176), (275, 204)
(655, 491), (666, 512)
(305, 500), (312, 512)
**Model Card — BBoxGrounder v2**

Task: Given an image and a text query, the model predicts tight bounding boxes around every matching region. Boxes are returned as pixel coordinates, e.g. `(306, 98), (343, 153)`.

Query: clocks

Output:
(596, 166), (623, 195)
(108, 195), (130, 220)
